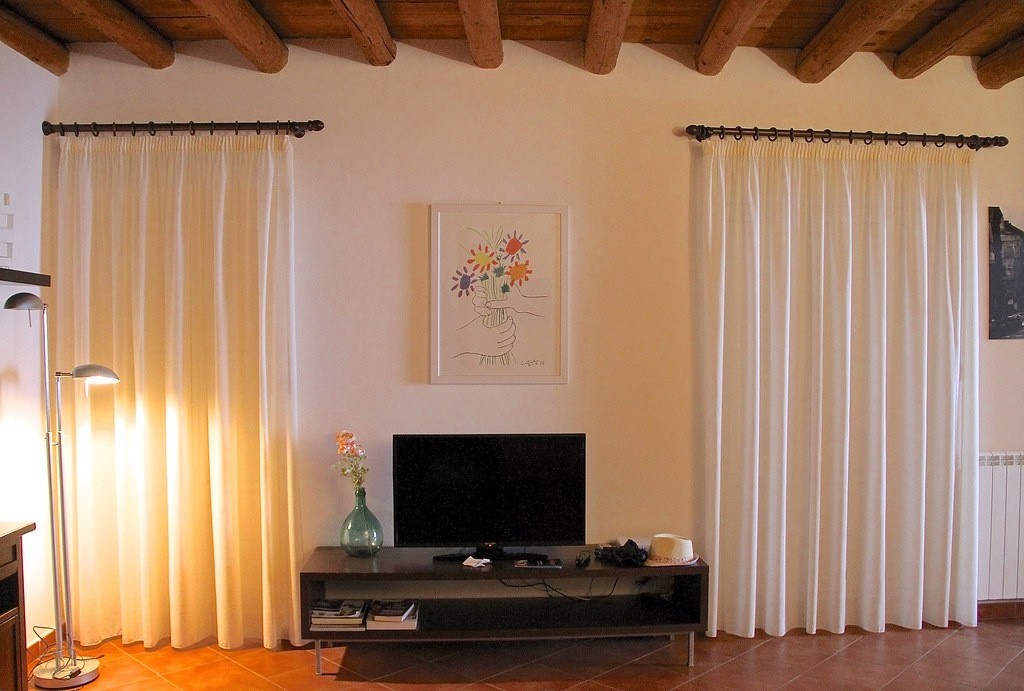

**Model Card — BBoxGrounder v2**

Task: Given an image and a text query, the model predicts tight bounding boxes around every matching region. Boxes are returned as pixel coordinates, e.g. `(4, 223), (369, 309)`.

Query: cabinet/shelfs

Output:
(0, 520), (37, 691)
(299, 546), (711, 676)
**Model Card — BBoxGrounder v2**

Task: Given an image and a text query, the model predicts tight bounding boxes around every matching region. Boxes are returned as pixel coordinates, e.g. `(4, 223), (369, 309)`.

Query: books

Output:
(308, 601), (366, 631)
(367, 599), (421, 630)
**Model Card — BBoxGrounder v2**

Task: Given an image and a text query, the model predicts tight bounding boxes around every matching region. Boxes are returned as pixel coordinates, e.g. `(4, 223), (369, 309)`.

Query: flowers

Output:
(332, 430), (373, 553)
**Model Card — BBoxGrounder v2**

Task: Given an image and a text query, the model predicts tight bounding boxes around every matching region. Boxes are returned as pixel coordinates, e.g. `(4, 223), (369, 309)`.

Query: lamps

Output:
(3, 291), (122, 689)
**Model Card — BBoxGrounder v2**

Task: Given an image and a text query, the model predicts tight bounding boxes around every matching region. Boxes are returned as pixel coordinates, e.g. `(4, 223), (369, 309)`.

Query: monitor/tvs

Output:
(392, 432), (586, 563)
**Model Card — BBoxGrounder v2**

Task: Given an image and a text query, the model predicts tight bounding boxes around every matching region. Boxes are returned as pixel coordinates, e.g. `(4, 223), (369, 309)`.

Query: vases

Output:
(340, 487), (384, 555)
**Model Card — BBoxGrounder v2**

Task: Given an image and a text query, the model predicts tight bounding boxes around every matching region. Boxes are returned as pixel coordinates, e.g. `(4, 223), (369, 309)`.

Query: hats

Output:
(644, 533), (700, 567)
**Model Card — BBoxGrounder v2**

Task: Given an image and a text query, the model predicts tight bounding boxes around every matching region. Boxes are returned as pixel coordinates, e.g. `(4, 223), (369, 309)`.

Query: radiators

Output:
(979, 450), (1024, 600)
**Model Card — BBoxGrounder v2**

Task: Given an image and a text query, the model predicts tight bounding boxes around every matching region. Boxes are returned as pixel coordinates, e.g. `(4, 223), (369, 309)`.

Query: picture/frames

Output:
(429, 202), (570, 385)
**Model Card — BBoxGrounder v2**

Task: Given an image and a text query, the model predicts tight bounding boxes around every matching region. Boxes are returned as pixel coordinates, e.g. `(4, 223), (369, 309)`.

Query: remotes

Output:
(576, 552), (590, 565)
